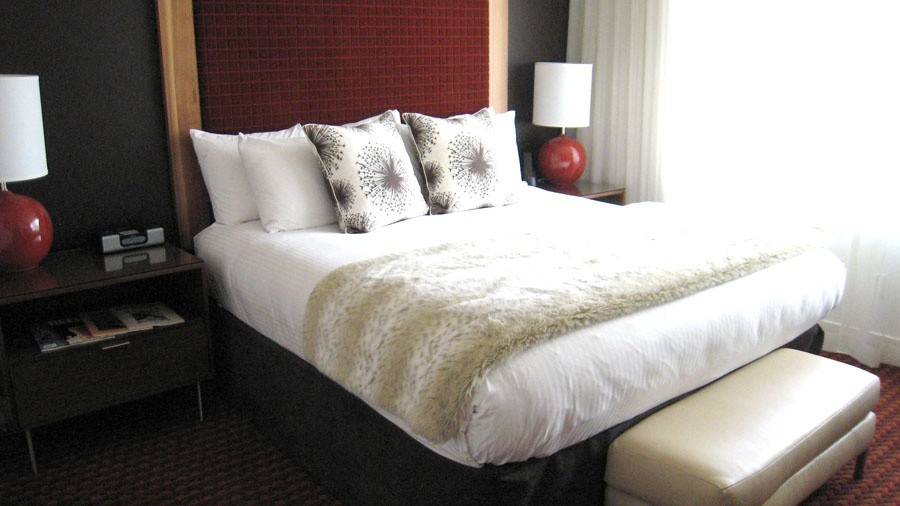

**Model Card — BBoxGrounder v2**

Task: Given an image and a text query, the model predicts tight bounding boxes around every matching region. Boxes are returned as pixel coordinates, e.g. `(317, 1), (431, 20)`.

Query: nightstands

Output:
(528, 180), (627, 206)
(0, 243), (214, 478)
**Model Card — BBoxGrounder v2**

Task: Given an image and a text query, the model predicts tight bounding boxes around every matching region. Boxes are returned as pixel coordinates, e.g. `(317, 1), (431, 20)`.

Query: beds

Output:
(155, 1), (846, 506)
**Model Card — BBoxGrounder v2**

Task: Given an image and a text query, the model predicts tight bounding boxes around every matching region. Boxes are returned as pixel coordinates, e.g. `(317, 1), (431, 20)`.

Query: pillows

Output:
(190, 122), (304, 227)
(239, 109), (427, 234)
(300, 109), (429, 234)
(402, 107), (522, 215)
(402, 107), (495, 202)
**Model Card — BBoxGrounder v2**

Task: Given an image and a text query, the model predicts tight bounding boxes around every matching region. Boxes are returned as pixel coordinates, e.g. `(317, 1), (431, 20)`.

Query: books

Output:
(30, 301), (185, 352)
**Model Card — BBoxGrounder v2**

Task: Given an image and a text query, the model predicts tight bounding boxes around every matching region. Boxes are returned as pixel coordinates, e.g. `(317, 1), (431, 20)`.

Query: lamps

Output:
(0, 73), (54, 272)
(531, 62), (594, 185)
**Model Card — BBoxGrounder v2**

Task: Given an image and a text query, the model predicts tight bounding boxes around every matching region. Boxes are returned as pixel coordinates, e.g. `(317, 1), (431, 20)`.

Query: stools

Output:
(603, 348), (881, 506)
